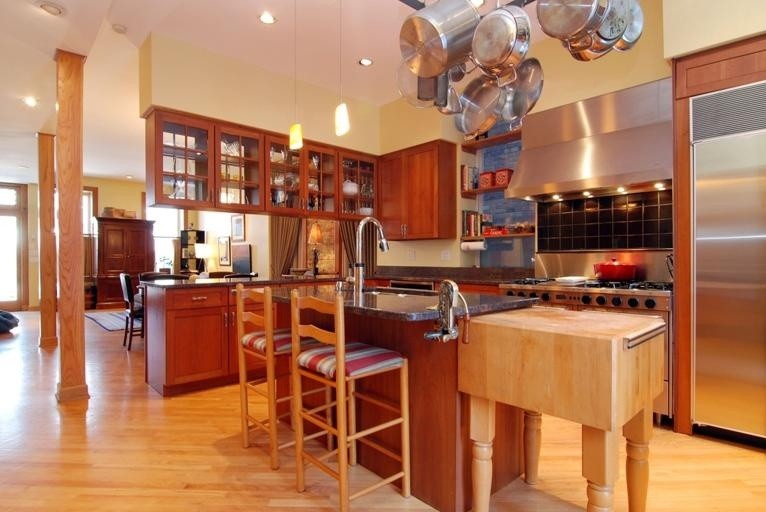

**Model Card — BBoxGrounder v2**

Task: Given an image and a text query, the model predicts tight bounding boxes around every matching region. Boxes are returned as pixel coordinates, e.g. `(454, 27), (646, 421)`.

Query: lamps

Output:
(308, 221), (323, 274)
(333, 0), (350, 137)
(36, 0), (127, 34)
(289, 0), (303, 150)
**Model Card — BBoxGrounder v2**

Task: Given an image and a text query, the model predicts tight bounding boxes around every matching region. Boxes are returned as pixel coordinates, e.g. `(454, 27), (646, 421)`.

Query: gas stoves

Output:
(501, 277), (673, 290)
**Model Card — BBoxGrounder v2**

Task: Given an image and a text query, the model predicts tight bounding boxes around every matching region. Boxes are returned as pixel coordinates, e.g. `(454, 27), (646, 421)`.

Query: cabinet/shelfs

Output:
(458, 126), (534, 241)
(142, 287), (277, 398)
(91, 216), (154, 303)
(174, 230), (204, 277)
(144, 108), (377, 222)
(378, 141), (455, 242)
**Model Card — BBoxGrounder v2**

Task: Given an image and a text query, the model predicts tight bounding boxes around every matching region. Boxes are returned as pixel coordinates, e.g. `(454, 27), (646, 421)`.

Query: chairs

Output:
(289, 281), (410, 512)
(120, 272), (143, 350)
(234, 283), (334, 469)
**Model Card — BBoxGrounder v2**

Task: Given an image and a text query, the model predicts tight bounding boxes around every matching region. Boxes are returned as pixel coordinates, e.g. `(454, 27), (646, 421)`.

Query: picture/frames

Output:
(217, 236), (231, 267)
(230, 215), (246, 242)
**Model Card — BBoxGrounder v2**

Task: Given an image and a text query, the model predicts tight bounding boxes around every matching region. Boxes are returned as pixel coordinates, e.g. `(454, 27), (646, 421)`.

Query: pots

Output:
(593, 257), (639, 281)
(398, 1), (644, 143)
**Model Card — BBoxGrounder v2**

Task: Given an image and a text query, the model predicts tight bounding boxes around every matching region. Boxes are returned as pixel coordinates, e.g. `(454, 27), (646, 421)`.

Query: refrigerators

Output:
(692, 128), (766, 447)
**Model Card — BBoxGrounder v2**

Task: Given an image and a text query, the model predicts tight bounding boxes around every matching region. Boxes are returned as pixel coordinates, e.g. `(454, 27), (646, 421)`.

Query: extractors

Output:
(503, 76), (672, 204)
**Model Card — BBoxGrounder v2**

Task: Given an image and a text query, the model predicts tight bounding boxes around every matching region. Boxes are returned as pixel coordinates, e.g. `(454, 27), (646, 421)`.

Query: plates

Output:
(555, 276), (588, 283)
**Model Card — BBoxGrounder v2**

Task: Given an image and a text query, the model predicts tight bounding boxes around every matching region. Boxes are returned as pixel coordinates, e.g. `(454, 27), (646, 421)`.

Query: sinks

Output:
(358, 286), (439, 297)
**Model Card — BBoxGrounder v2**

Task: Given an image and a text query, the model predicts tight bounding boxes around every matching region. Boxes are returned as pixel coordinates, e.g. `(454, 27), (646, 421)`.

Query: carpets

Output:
(85, 309), (142, 331)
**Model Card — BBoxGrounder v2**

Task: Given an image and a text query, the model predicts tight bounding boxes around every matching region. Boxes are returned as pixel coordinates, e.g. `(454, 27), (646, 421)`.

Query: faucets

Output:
(354, 216), (389, 292)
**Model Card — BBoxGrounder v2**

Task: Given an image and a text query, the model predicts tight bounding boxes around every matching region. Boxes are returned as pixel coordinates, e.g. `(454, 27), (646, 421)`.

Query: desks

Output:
(459, 306), (666, 512)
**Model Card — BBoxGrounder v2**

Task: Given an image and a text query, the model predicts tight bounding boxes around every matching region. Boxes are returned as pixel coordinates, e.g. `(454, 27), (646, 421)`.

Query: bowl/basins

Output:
(342, 180), (358, 196)
(360, 207), (373, 216)
